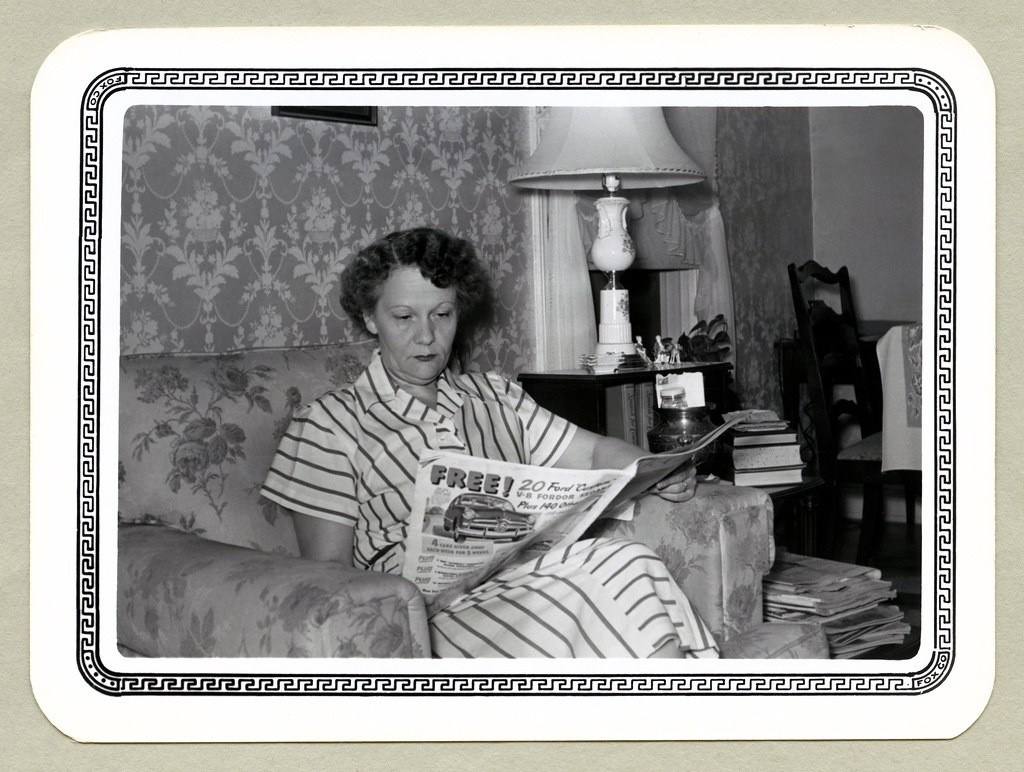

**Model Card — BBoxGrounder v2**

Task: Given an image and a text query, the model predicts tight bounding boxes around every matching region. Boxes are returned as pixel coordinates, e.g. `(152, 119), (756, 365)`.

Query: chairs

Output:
(789, 258), (922, 607)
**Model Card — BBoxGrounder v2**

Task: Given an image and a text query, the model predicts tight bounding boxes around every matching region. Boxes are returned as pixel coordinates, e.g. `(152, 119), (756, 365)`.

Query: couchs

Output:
(117, 334), (828, 658)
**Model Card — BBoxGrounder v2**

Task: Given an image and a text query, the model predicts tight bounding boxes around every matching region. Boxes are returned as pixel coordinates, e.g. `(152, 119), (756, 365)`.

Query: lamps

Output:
(506, 107), (707, 364)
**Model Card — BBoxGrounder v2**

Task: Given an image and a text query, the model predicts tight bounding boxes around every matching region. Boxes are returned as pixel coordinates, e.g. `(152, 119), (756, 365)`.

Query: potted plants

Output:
(646, 314), (733, 476)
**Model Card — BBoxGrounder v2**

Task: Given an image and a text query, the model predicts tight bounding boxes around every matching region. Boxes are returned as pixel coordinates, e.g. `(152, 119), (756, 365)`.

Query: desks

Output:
(875, 323), (924, 473)
(518, 361), (735, 483)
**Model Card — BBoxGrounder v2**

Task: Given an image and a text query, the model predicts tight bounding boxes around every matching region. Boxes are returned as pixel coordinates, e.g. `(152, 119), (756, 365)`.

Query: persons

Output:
(257, 228), (721, 659)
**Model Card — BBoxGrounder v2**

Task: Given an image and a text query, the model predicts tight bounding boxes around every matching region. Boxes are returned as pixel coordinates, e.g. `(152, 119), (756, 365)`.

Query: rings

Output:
(682, 480), (688, 491)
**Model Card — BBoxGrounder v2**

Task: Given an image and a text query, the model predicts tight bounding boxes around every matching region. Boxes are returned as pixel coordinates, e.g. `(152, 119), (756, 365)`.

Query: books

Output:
(716, 422), (807, 487)
(762, 551), (912, 659)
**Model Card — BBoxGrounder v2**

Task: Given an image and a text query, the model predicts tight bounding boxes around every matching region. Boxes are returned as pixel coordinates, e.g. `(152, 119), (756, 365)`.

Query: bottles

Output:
(660, 387), (688, 409)
